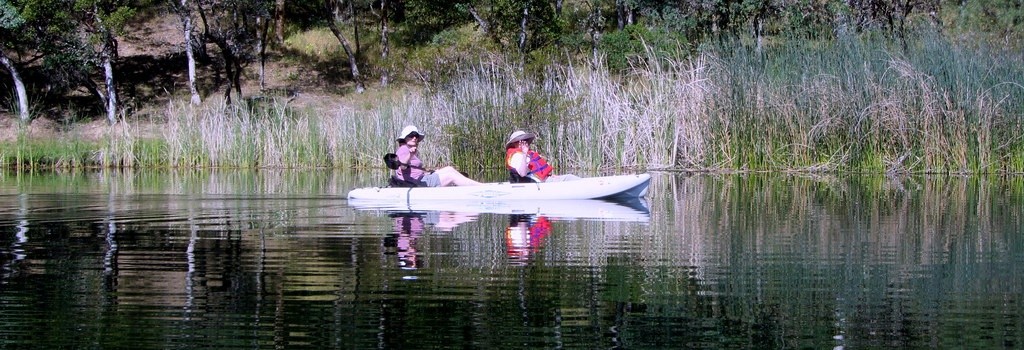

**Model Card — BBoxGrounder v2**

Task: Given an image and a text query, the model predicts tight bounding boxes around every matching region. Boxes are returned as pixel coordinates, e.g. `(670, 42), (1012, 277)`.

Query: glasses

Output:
(408, 133), (420, 138)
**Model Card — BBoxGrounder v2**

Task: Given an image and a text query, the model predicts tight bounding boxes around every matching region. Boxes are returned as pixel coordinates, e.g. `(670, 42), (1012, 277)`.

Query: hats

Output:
(396, 125), (425, 142)
(507, 131), (535, 145)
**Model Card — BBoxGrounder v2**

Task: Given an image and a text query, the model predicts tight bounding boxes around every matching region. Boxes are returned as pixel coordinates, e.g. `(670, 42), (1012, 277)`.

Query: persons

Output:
(505, 131), (580, 184)
(396, 125), (482, 187)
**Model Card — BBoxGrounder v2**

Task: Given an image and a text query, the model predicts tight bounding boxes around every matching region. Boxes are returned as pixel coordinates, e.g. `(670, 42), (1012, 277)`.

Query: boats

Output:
(348, 199), (651, 222)
(346, 173), (650, 199)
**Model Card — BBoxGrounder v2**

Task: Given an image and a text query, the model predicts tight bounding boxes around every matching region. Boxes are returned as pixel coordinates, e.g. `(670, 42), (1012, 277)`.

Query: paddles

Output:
(383, 153), (470, 179)
